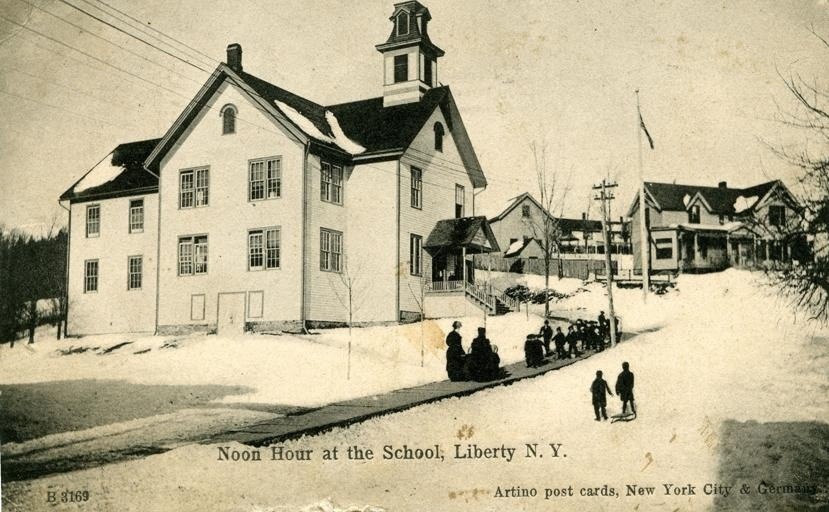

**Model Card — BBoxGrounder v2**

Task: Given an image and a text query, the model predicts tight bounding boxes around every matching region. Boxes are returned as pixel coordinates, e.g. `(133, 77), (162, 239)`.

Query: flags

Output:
(639, 107), (656, 149)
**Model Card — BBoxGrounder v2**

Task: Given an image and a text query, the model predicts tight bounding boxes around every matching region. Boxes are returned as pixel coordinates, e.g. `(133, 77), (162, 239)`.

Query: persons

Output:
(590, 362), (637, 421)
(443, 321), (502, 382)
(524, 311), (620, 368)
